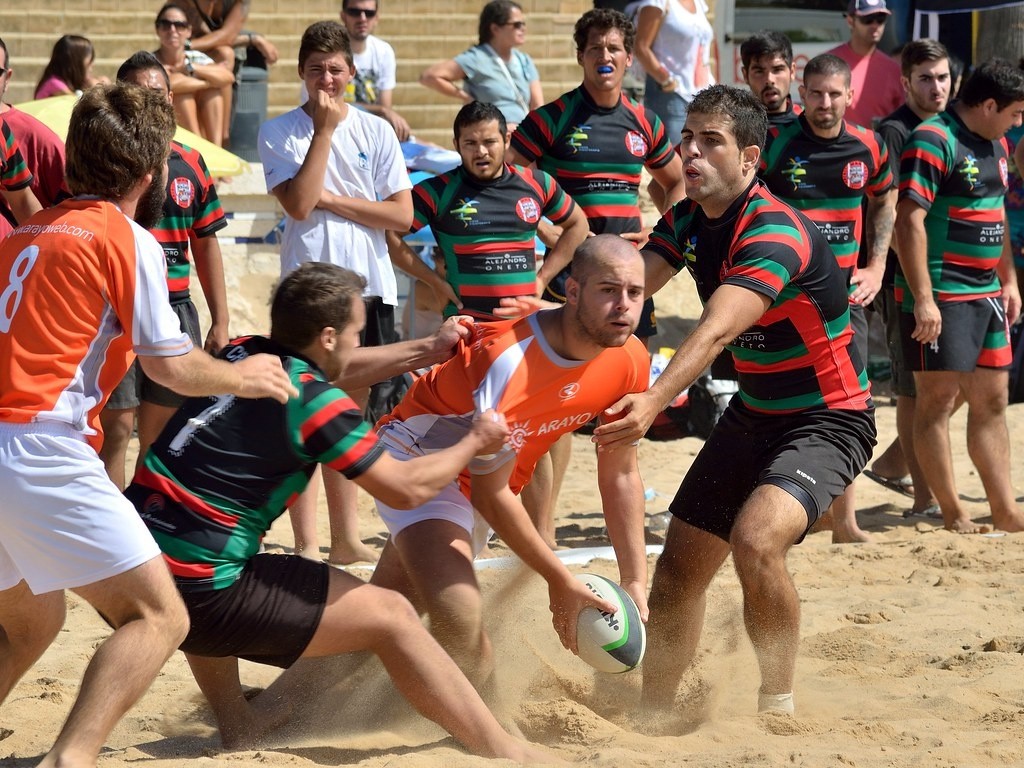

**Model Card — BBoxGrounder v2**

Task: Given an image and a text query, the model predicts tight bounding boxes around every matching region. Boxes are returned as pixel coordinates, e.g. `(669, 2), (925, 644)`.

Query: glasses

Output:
(500, 21), (526, 29)
(0, 69), (7, 76)
(341, 7), (378, 18)
(859, 12), (887, 25)
(154, 20), (190, 33)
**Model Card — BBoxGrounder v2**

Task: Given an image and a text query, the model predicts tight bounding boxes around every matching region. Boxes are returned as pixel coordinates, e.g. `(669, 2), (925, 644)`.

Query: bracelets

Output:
(661, 77), (673, 87)
(249, 34), (253, 46)
(186, 63), (193, 77)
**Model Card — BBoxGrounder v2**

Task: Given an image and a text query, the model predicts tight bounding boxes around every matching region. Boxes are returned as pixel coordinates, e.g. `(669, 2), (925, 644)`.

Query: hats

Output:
(847, 0), (895, 15)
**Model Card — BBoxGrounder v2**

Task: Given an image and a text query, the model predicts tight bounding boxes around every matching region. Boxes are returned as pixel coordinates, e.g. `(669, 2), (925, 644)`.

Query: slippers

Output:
(862, 468), (917, 499)
(902, 504), (945, 520)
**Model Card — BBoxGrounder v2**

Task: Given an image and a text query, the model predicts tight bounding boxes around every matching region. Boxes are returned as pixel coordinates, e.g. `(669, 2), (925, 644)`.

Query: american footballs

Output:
(568, 573), (647, 674)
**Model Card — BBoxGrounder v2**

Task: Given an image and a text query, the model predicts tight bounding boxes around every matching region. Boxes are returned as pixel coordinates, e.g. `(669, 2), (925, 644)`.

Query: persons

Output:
(0, 38), (73, 245)
(862, 39), (952, 519)
(369, 233), (649, 696)
(98, 52), (230, 491)
(898, 57), (1024, 532)
(168, 0), (279, 148)
(635, 0), (715, 146)
(337, 0), (410, 143)
(388, 100), (590, 550)
(257, 21), (414, 565)
(509, 10), (688, 551)
(758, 54), (898, 543)
(122, 263), (530, 759)
(151, 4), (236, 149)
(827, 0), (907, 130)
(420, 0), (544, 135)
(739, 29), (806, 127)
(493, 84), (878, 717)
(33, 33), (110, 101)
(0, 82), (299, 768)
